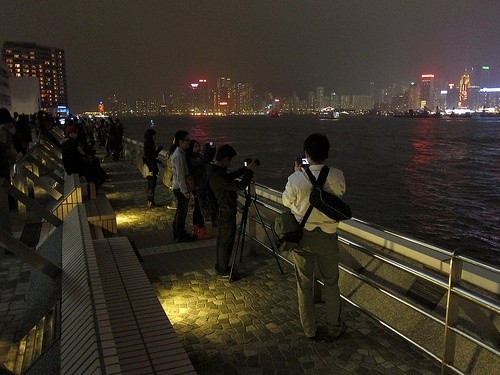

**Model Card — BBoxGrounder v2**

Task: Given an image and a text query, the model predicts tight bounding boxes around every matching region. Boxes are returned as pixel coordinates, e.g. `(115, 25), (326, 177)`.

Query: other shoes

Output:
(329, 321), (346, 338)
(167, 204), (177, 209)
(176, 232), (191, 242)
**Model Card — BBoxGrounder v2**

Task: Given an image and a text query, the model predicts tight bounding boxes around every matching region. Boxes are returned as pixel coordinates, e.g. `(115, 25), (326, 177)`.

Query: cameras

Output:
(302, 158), (309, 165)
(244, 158), (260, 168)
(209, 141), (214, 145)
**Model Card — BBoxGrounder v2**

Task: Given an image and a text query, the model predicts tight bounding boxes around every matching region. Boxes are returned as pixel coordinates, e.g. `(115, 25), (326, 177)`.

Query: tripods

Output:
(230, 184), (284, 284)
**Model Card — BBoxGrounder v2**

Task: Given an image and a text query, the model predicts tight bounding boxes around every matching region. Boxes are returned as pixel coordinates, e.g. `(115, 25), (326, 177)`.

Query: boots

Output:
(193, 224), (199, 237)
(198, 228), (213, 240)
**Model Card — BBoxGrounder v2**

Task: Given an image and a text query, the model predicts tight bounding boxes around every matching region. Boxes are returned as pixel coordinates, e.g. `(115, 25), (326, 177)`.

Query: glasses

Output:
(183, 139), (190, 142)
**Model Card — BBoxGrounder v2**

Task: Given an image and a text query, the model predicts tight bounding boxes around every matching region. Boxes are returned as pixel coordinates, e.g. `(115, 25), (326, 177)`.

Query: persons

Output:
(0, 108), (258, 276)
(283, 133), (346, 340)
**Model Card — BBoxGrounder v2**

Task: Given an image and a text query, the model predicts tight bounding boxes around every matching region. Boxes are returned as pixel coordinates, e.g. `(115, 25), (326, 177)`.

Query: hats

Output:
(66, 126), (75, 136)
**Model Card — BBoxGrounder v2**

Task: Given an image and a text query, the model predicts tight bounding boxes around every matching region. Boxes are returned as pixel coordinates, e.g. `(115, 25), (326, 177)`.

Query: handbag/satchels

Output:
(137, 157), (149, 178)
(272, 212), (302, 252)
(303, 166), (352, 221)
(162, 170), (173, 188)
(186, 174), (194, 193)
